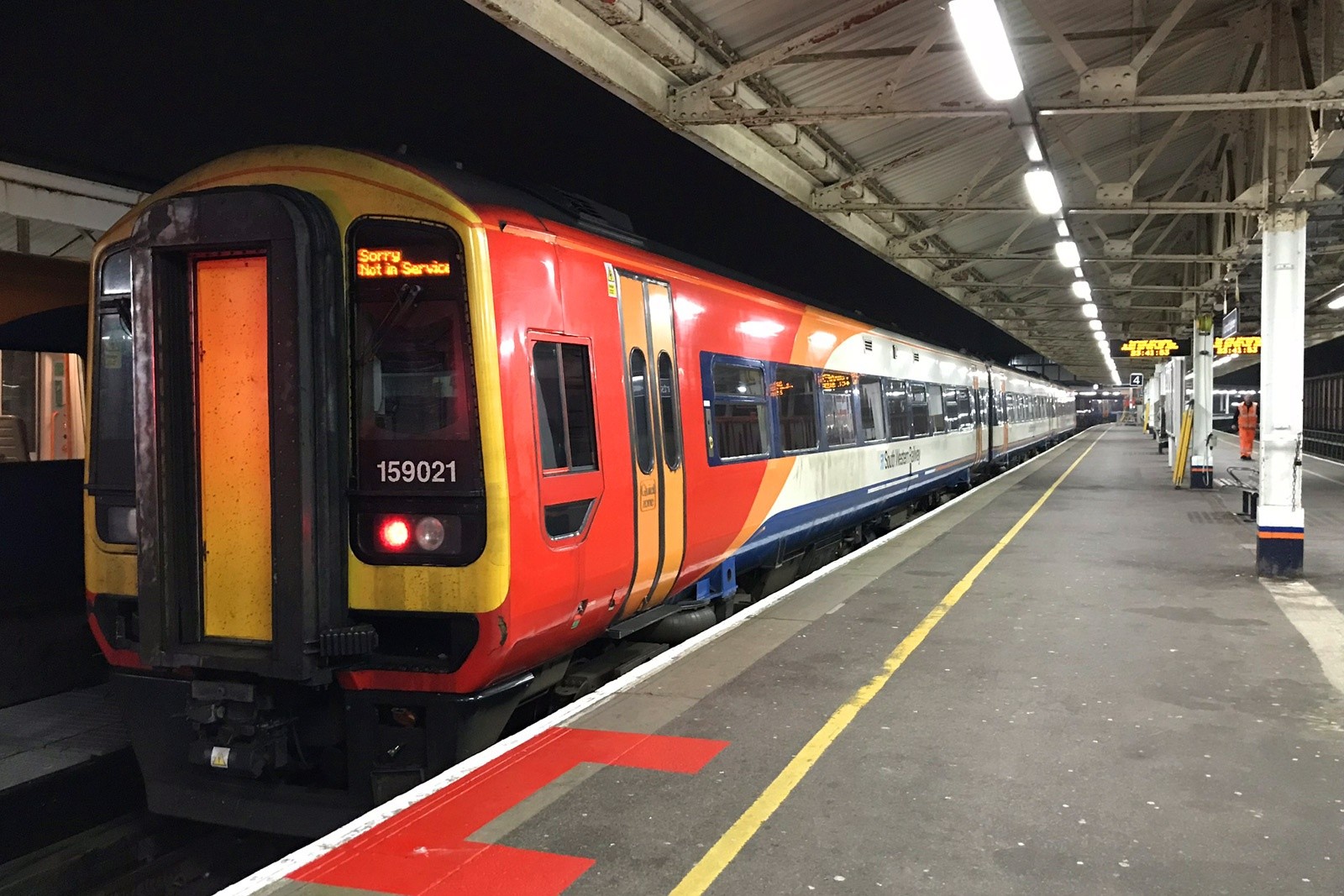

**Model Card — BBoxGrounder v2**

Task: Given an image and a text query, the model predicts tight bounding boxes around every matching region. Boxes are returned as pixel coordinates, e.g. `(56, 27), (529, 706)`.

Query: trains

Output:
(80, 143), (1125, 838)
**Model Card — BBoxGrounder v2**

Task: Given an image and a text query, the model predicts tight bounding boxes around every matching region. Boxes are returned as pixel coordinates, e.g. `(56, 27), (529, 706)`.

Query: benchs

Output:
(1213, 463), (1259, 523)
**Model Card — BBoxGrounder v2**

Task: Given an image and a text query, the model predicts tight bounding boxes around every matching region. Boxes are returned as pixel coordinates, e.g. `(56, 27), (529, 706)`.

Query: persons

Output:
(1231, 393), (1260, 460)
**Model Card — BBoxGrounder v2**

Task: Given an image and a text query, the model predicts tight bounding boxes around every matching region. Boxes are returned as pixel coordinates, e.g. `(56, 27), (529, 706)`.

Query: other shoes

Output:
(1240, 455), (1252, 461)
